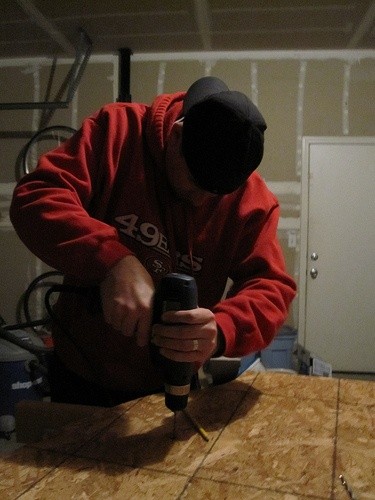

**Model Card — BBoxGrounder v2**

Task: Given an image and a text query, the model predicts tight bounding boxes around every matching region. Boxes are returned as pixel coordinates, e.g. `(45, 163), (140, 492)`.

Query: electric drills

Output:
(77, 271), (199, 440)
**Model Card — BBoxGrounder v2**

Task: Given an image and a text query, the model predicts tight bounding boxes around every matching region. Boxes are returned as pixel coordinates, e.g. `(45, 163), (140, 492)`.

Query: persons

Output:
(9, 76), (297, 407)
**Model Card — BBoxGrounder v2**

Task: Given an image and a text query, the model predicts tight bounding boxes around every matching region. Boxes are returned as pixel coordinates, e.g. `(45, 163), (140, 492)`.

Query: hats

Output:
(183, 76), (268, 195)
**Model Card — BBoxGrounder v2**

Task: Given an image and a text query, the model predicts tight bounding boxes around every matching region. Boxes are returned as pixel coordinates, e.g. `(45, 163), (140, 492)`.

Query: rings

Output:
(194, 339), (198, 351)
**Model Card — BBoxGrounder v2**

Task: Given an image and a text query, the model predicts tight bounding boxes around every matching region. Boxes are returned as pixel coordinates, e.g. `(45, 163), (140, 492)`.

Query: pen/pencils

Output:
(339, 474), (356, 499)
(181, 406), (210, 442)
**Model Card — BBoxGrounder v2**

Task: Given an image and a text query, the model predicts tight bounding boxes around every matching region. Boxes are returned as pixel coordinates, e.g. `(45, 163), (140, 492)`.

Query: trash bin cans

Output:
(0, 323), (46, 442)
(258, 325), (298, 374)
